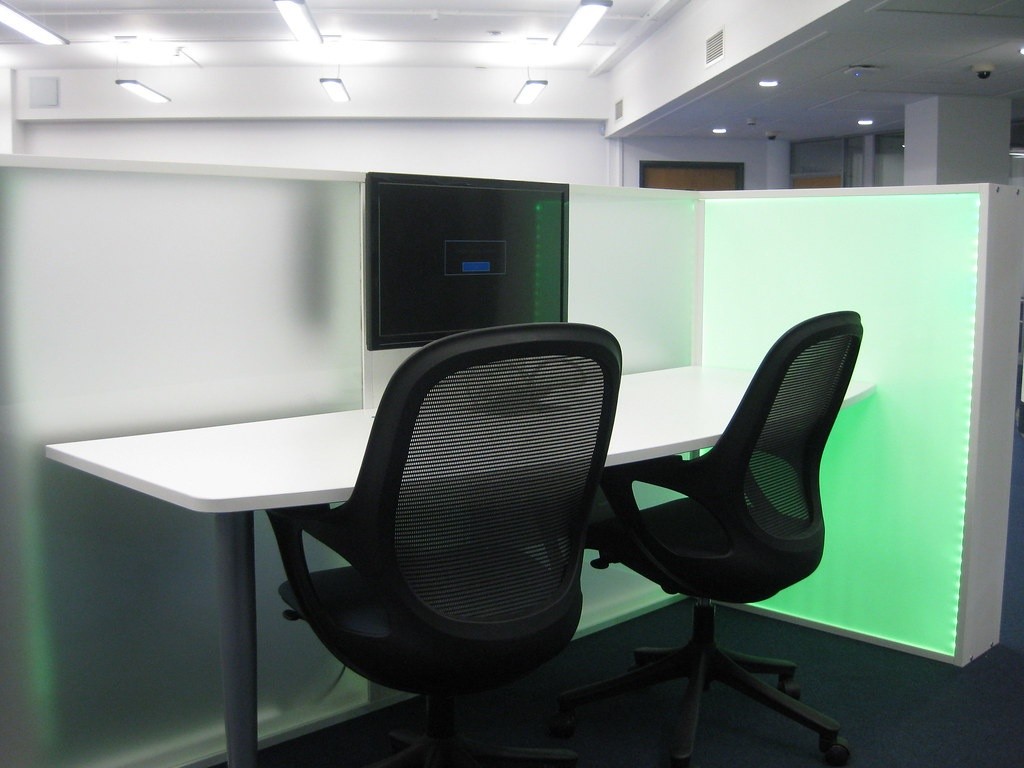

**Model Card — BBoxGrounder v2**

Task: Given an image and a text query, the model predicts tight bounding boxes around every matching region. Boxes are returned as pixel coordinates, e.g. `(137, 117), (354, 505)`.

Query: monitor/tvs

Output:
(365, 173), (569, 351)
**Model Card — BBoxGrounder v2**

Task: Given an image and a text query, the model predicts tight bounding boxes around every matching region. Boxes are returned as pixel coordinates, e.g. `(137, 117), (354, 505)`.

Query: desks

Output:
(43, 355), (876, 768)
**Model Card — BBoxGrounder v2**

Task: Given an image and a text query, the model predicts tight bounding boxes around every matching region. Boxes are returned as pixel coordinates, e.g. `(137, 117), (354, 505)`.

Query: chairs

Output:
(584, 310), (865, 768)
(270, 321), (624, 768)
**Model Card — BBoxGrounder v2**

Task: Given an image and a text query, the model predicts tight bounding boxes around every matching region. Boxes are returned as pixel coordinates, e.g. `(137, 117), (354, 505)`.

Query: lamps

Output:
(0, 0), (612, 109)
